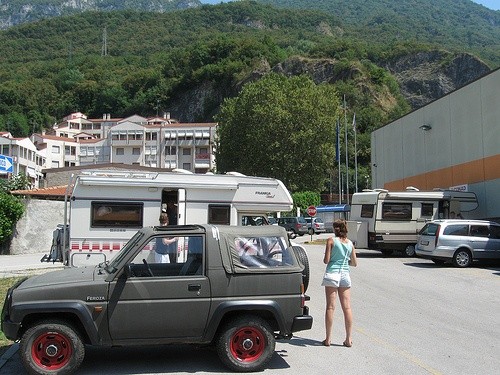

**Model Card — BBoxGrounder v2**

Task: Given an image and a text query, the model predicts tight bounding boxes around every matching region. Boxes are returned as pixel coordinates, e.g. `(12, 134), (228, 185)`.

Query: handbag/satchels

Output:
(320, 272), (341, 288)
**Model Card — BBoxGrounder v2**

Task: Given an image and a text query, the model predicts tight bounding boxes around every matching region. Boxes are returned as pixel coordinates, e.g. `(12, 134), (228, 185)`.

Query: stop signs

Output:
(306, 206), (317, 216)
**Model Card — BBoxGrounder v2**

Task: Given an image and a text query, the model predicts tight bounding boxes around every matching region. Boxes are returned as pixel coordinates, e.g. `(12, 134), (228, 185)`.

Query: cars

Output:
(414, 219), (500, 267)
(253, 216), (325, 236)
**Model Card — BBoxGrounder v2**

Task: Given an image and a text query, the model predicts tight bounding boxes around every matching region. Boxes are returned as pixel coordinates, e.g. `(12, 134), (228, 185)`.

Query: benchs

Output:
(241, 244), (285, 266)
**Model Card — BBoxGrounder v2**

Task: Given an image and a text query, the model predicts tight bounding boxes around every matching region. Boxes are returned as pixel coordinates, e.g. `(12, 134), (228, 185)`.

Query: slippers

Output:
(343, 340), (352, 347)
(323, 339), (330, 345)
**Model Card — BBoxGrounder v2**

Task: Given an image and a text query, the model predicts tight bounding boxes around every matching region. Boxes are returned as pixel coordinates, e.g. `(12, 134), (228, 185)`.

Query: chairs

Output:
(179, 238), (202, 276)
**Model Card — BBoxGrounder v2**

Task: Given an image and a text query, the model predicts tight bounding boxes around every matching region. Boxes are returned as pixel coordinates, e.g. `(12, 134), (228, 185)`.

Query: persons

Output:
(321, 219), (357, 347)
(154, 218), (178, 263)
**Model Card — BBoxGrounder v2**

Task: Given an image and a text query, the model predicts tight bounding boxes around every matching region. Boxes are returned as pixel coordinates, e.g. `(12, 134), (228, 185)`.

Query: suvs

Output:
(1, 224), (312, 374)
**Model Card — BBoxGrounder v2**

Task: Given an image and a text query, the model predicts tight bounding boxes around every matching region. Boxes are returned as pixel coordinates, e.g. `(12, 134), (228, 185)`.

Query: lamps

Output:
(419, 125), (432, 131)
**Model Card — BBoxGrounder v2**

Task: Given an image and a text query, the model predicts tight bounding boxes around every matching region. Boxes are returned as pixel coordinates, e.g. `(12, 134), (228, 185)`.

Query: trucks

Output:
(39, 169), (293, 268)
(343, 186), (479, 257)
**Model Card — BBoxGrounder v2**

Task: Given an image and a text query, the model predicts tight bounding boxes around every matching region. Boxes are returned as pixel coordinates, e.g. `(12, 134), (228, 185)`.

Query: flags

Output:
(352, 113), (356, 131)
